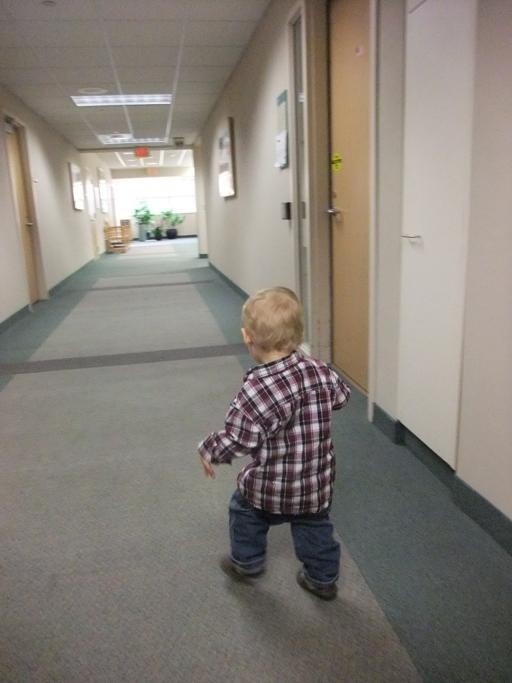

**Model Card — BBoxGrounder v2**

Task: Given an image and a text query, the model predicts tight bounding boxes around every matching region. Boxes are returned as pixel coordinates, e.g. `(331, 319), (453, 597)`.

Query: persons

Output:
(196, 287), (352, 602)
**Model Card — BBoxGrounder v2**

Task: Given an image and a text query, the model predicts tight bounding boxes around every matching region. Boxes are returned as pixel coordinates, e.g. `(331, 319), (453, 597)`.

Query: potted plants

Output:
(132, 204), (184, 244)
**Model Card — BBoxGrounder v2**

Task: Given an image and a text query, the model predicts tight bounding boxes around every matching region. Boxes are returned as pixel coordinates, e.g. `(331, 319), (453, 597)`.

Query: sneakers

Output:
(221, 558), (264, 582)
(298, 571), (337, 599)
